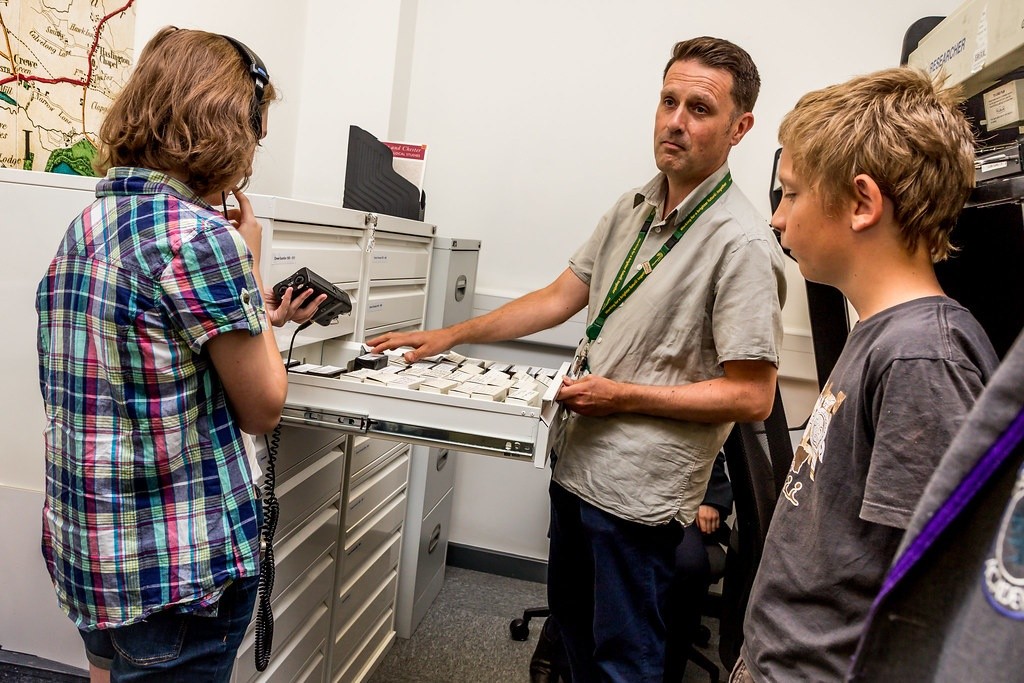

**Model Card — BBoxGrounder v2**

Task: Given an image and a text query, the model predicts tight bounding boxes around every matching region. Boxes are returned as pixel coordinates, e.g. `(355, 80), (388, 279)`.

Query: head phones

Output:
(220, 34), (270, 113)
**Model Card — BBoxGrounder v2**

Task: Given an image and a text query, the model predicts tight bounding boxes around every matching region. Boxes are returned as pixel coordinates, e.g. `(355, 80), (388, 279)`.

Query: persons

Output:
(366, 34), (786, 683)
(35, 25), (329, 683)
(720, 69), (1024, 683)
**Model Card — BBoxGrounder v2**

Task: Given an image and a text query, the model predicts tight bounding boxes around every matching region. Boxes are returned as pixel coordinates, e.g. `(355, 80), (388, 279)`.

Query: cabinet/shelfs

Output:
(0, 165), (577, 683)
(394, 236), (481, 632)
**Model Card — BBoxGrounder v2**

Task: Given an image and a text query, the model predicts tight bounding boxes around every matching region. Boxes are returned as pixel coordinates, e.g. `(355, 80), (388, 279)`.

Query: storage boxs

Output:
(342, 125), (427, 221)
